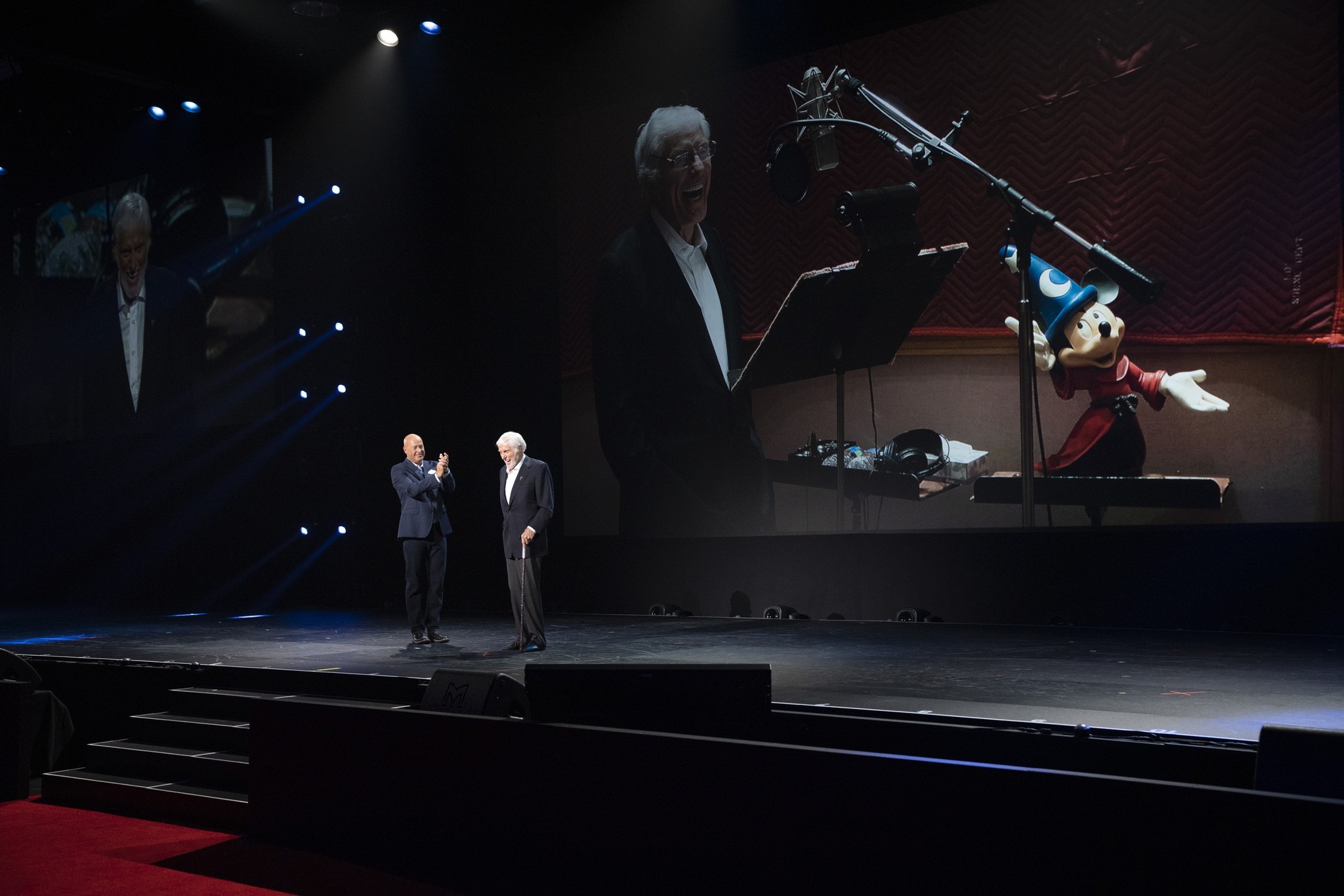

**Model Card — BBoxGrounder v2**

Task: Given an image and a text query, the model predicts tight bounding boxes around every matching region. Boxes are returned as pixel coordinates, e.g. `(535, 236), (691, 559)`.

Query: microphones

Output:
(802, 66), (840, 171)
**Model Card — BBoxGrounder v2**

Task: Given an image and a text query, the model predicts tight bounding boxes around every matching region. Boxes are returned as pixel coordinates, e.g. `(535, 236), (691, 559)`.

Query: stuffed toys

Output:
(998, 241), (1230, 478)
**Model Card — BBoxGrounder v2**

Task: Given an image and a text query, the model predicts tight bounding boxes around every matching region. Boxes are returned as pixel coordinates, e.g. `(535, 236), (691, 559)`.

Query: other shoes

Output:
(523, 644), (538, 652)
(502, 640), (526, 650)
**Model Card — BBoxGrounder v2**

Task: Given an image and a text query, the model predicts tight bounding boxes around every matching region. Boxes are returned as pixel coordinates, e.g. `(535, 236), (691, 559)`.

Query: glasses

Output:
(647, 139), (717, 171)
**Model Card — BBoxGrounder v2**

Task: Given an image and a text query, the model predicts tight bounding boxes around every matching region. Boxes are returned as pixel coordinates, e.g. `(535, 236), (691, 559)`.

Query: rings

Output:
(528, 541), (530, 542)
(445, 461), (447, 463)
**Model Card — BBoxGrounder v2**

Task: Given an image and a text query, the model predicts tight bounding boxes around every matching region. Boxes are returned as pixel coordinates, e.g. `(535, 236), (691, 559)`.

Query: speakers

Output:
(1252, 723), (1344, 802)
(421, 667), (530, 720)
(0, 648), (42, 685)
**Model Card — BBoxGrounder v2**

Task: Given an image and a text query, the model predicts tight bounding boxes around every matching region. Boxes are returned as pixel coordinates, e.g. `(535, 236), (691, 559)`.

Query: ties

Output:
(420, 467), (425, 479)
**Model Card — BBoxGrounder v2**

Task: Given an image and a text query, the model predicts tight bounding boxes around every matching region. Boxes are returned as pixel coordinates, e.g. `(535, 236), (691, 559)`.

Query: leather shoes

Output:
(412, 630), (431, 643)
(427, 629), (450, 642)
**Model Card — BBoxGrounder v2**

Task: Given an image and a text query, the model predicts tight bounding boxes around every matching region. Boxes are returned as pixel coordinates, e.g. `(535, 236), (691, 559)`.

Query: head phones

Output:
(874, 428), (951, 485)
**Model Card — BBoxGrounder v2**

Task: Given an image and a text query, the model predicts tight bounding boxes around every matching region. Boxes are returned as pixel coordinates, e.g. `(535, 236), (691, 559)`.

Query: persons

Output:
(390, 433), (456, 644)
(496, 431), (555, 652)
(588, 103), (776, 538)
(74, 192), (209, 441)
